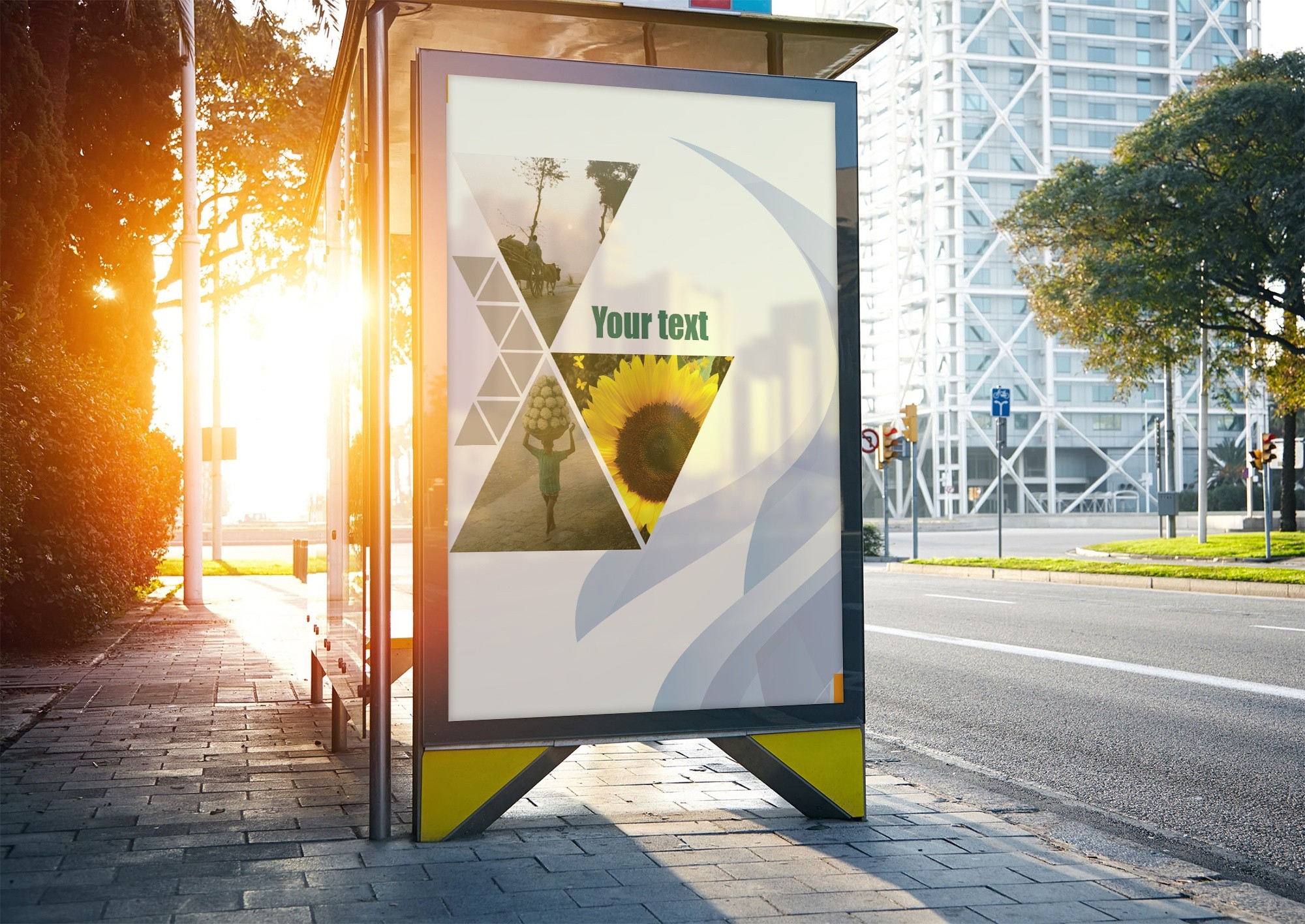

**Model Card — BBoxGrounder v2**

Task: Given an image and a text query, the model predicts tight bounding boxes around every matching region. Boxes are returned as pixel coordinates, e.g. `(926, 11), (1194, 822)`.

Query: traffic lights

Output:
(899, 405), (918, 443)
(879, 425), (898, 463)
(1262, 433), (1276, 464)
(1249, 449), (1262, 470)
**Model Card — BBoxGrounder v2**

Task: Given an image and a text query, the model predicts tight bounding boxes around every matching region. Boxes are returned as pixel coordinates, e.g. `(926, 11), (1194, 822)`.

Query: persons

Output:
(528, 235), (542, 262)
(522, 423), (575, 541)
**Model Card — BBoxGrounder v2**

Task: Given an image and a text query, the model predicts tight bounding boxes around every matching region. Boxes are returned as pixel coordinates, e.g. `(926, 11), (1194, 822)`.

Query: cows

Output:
(542, 264), (561, 295)
